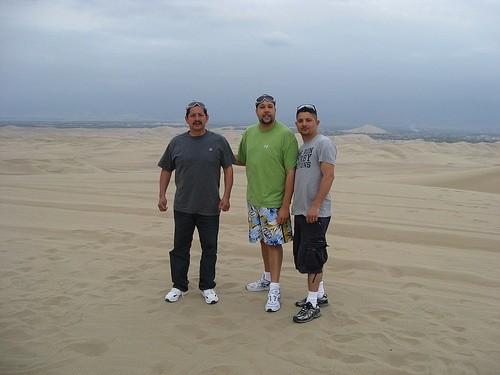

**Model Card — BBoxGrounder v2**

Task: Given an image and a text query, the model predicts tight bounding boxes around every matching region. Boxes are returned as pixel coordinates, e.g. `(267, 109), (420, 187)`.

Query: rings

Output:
(226, 209), (228, 211)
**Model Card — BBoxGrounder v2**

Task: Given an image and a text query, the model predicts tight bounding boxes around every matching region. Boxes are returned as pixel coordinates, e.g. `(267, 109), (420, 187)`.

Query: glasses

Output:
(297, 104), (315, 111)
(186, 102), (206, 109)
(255, 96), (275, 103)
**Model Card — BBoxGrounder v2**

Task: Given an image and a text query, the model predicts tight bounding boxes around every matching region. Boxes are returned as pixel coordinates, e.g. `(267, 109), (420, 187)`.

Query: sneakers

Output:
(165, 287), (188, 302)
(245, 275), (271, 292)
(296, 294), (329, 307)
(202, 289), (219, 303)
(265, 288), (281, 312)
(293, 299), (321, 323)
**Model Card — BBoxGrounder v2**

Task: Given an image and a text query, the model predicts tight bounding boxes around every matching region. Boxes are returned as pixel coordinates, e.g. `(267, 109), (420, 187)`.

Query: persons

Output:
(157, 102), (236, 304)
(232, 94), (298, 311)
(291, 103), (338, 323)
